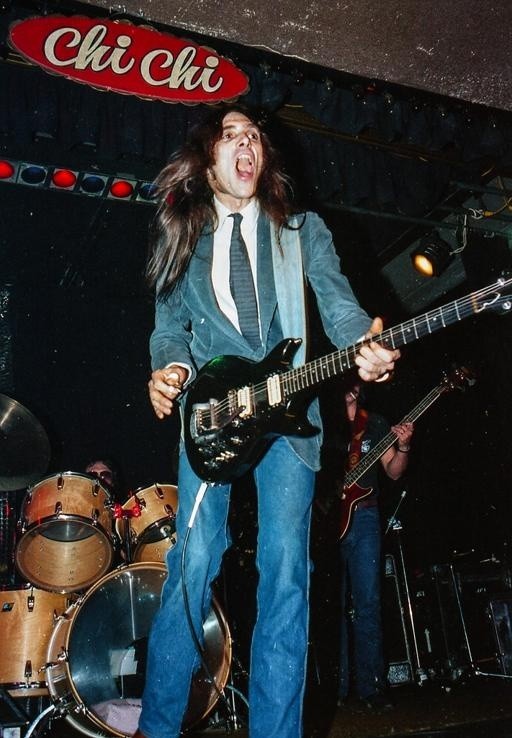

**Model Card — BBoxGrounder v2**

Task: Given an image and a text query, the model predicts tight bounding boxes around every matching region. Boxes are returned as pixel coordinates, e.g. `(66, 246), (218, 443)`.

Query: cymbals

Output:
(0, 394), (50, 492)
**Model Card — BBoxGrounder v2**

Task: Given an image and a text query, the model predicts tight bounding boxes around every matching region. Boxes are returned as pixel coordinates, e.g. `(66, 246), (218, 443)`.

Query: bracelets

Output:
(398, 446), (410, 453)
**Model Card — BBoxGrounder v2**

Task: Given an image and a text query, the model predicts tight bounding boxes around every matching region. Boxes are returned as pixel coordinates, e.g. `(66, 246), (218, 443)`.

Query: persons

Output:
(140, 106), (401, 737)
(86, 455), (119, 488)
(314, 383), (415, 710)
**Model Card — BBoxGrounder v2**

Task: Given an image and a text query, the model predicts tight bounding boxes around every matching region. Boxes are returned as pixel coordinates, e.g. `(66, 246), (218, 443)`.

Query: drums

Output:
(14, 469), (117, 594)
(2, 580), (79, 699)
(47, 560), (232, 738)
(118, 480), (179, 563)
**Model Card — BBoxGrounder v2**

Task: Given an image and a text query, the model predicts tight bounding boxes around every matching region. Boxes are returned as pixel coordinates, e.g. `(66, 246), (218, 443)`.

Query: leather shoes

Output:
(362, 692), (395, 713)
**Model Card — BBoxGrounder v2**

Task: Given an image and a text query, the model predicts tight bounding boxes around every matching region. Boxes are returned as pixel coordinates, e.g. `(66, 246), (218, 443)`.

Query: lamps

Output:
(410, 213), (469, 276)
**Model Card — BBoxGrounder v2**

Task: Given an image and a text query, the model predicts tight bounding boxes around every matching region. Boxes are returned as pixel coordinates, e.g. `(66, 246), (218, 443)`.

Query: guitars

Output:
(314, 363), (475, 551)
(185, 278), (509, 484)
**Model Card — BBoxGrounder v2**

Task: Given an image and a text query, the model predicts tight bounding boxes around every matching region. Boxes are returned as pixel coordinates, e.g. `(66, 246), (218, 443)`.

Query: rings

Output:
(165, 373), (179, 381)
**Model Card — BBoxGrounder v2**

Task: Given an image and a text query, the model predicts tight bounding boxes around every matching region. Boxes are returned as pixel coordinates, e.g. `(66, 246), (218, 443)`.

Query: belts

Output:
(350, 497), (378, 511)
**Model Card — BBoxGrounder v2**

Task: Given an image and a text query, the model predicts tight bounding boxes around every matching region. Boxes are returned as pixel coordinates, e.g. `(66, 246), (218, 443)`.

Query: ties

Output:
(226, 213), (264, 353)
